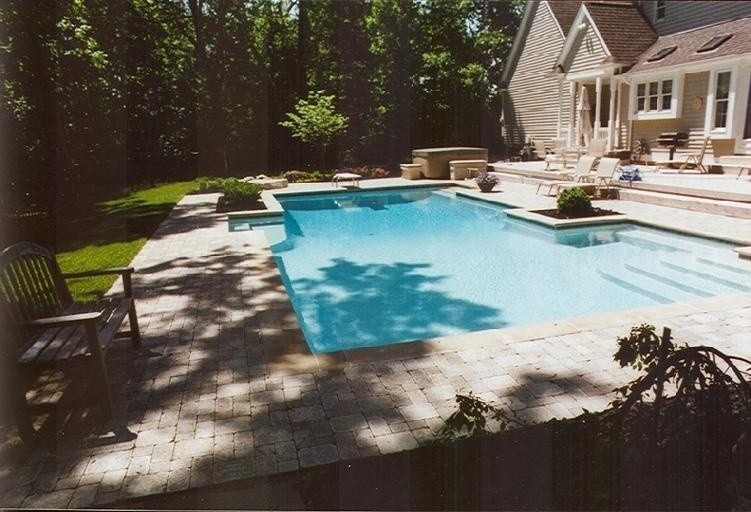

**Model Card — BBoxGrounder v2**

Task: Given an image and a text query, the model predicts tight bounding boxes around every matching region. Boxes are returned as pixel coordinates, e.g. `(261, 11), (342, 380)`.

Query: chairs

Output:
(535, 133), (751, 199)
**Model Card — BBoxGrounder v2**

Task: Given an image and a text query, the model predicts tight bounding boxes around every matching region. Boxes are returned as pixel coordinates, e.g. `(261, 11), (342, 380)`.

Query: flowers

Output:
(476, 164), (500, 183)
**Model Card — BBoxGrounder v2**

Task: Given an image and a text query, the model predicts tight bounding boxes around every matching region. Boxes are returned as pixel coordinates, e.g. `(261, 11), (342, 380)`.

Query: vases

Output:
(478, 181), (498, 192)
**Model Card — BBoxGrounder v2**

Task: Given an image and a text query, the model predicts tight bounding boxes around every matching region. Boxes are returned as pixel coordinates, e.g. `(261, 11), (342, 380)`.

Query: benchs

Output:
(0, 238), (140, 432)
